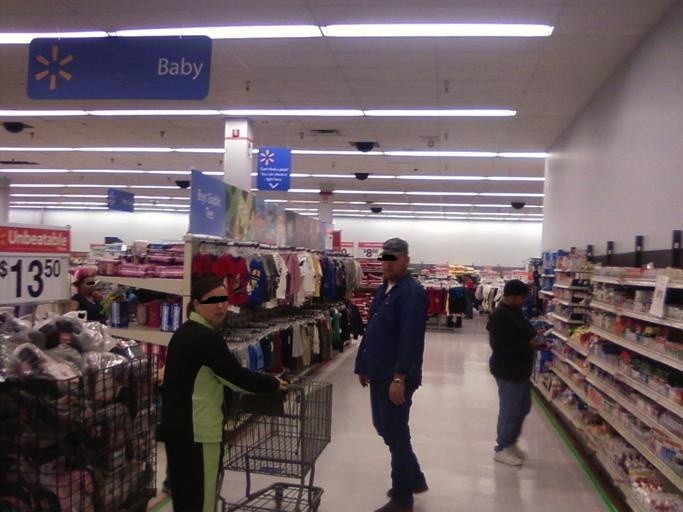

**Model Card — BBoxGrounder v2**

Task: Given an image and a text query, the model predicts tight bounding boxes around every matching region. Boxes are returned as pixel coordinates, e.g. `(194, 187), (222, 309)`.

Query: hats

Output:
(378, 238), (408, 256)
(71, 266), (98, 287)
(503, 279), (529, 295)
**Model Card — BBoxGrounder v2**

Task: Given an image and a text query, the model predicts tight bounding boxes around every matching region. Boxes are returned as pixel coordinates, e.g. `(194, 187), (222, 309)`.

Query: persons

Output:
(353, 238), (429, 512)
(158, 271), (288, 512)
(464, 274), (474, 288)
(533, 271), (541, 287)
(486, 279), (544, 466)
(72, 275), (108, 324)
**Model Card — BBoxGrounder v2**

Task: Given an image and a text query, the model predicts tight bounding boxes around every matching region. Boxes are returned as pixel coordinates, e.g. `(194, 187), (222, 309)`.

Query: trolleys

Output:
(217, 370), (332, 511)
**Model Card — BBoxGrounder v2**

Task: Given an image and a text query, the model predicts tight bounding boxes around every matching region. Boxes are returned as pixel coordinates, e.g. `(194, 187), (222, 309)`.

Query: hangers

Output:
(419, 261), (530, 300)
(217, 301), (359, 358)
(193, 239), (361, 267)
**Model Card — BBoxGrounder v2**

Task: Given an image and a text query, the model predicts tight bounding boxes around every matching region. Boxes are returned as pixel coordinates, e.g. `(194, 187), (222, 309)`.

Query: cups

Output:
(108, 301), (129, 328)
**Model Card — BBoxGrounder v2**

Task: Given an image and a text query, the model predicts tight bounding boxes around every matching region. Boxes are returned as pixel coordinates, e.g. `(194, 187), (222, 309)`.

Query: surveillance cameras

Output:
(175, 179), (192, 188)
(355, 140), (376, 152)
(3, 120), (25, 133)
(354, 171), (369, 180)
(370, 207), (384, 213)
(511, 201), (525, 209)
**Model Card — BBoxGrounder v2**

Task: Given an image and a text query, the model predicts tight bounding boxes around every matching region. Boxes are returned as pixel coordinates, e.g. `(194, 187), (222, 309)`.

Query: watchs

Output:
(393, 378), (404, 384)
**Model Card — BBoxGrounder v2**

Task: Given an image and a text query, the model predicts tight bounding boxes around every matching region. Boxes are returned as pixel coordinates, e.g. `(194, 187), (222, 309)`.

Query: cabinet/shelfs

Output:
(95, 275), (183, 442)
(352, 267), (384, 324)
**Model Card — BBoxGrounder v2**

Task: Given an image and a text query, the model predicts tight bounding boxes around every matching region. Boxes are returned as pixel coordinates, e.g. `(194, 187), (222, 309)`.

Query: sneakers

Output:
(493, 439), (528, 467)
(374, 502), (414, 512)
(387, 472), (429, 498)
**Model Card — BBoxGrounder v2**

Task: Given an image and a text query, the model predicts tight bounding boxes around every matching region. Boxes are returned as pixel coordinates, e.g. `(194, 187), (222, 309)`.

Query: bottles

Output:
(601, 326), (683, 512)
(570, 273), (593, 320)
